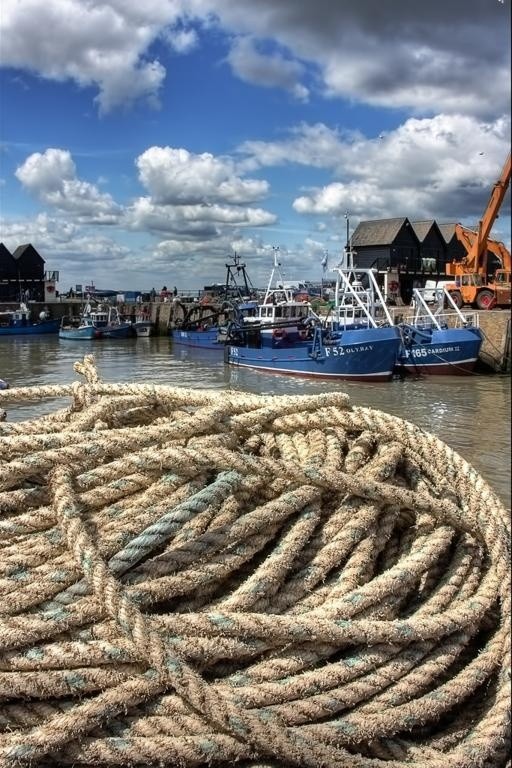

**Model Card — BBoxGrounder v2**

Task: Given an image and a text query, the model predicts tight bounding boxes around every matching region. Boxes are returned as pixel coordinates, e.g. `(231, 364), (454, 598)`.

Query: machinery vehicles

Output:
(442, 150), (512, 310)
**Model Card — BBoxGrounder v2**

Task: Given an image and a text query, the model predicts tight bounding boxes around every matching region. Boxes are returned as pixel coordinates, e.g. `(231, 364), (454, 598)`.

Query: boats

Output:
(222, 248), (402, 384)
(0, 294), (156, 342)
(317, 218), (484, 378)
(170, 249), (260, 351)
